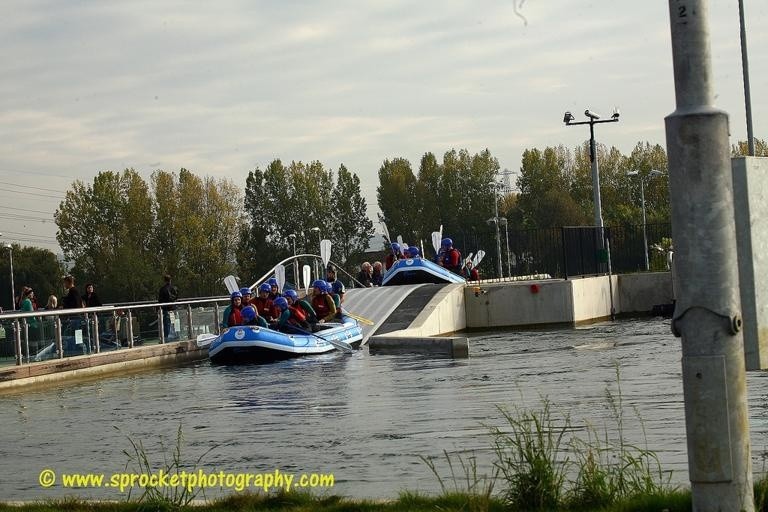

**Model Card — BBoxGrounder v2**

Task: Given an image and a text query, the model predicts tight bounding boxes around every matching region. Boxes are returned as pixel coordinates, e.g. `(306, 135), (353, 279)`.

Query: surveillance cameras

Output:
(584, 110), (600, 118)
(613, 106), (620, 119)
(563, 112), (571, 123)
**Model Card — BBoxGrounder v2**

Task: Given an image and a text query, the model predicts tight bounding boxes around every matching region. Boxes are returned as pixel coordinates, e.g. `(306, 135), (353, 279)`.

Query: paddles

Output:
(381, 221), (485, 273)
(314, 324), (348, 327)
(303, 265), (311, 302)
(274, 264), (285, 297)
(320, 239), (331, 292)
(196, 334), (221, 347)
(285, 323), (352, 352)
(341, 311), (374, 325)
(224, 275), (240, 294)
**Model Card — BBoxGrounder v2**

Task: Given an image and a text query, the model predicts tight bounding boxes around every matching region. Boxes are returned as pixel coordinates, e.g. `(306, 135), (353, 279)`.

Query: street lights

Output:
(624, 168), (669, 271)
(287, 232), (298, 292)
(485, 181), (506, 279)
(311, 226), (324, 280)
(2, 244), (17, 309)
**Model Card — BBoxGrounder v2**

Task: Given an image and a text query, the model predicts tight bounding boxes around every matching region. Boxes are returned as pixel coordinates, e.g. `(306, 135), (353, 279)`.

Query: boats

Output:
(160, 317), (206, 342)
(208, 315), (364, 363)
(380, 259), (466, 284)
(33, 338), (118, 361)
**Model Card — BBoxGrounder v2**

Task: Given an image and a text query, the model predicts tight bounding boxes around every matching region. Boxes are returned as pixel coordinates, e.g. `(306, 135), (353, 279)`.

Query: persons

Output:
(385, 237), (482, 282)
(219, 263), (346, 335)
(353, 260), (375, 290)
(157, 273), (178, 344)
(110, 306), (130, 349)
(17, 275), (101, 362)
(370, 261), (384, 287)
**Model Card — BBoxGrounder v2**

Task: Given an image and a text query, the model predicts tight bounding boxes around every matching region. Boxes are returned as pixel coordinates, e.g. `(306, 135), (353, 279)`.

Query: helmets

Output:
(325, 265), (338, 274)
(272, 297), (288, 311)
(312, 280), (333, 294)
(258, 283), (272, 294)
(390, 243), (420, 255)
(231, 291), (242, 299)
(282, 289), (298, 302)
(240, 306), (257, 323)
(239, 288), (253, 295)
(441, 238), (453, 248)
